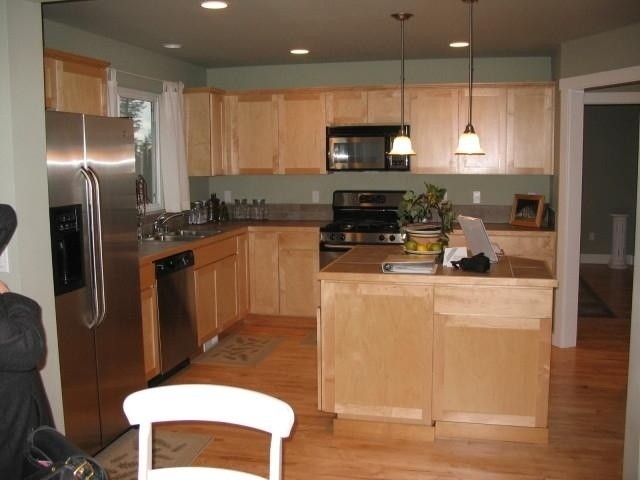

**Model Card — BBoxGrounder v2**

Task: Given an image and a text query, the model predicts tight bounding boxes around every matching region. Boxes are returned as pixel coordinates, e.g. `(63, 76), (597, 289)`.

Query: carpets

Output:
(575, 271), (619, 320)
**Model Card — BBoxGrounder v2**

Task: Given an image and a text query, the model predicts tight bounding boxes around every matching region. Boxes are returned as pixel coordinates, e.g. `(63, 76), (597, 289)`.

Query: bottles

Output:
(187, 193), (229, 223)
(230, 197), (271, 219)
(136, 171), (147, 207)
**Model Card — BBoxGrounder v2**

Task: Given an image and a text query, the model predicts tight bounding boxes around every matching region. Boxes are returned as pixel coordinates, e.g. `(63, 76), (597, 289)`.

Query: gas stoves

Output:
(320, 217), (406, 245)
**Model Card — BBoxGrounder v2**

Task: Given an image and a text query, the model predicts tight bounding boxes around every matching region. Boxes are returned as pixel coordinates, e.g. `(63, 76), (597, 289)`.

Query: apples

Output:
(431, 244), (440, 251)
(405, 240), (417, 250)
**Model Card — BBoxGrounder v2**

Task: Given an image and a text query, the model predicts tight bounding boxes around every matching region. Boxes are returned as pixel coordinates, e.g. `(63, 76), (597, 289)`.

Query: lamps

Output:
(454, 0), (486, 155)
(387, 12), (419, 157)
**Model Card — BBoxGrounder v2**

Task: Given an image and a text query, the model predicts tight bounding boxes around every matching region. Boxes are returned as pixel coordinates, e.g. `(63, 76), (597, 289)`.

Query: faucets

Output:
(163, 211), (187, 224)
(156, 212), (168, 221)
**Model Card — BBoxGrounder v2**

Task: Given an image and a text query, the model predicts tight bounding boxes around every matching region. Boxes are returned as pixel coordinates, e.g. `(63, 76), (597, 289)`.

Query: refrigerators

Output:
(46, 111), (146, 465)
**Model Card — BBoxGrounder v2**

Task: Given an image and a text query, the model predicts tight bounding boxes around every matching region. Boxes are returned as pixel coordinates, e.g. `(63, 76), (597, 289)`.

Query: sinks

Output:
(167, 229), (223, 236)
(144, 234), (206, 242)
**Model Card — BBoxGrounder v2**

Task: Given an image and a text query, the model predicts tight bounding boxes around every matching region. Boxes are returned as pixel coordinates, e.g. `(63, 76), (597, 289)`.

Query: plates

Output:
(405, 246), (442, 256)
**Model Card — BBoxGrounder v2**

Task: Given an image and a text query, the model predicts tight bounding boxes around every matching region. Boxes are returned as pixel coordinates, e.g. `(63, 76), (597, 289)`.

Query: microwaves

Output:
(325, 125), (408, 171)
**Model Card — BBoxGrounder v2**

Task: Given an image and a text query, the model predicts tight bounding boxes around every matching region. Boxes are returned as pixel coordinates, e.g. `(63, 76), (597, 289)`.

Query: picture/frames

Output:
(508, 192), (546, 229)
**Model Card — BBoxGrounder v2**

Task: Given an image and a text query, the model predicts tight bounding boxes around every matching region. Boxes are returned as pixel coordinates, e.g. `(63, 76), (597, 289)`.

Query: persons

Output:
(0, 204), (57, 479)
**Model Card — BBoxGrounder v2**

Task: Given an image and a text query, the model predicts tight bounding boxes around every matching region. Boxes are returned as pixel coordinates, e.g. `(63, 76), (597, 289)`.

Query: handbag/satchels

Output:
(23, 426), (110, 480)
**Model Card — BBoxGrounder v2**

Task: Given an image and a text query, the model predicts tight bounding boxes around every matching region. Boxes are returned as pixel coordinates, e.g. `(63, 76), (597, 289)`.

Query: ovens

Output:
(318, 245), (355, 272)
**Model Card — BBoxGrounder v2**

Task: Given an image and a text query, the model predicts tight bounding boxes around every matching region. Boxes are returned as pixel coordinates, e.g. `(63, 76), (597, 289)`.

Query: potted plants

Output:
(397, 180), (456, 249)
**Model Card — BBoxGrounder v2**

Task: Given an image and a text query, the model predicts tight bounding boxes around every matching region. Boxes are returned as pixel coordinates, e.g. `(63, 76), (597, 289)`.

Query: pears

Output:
(417, 245), (427, 251)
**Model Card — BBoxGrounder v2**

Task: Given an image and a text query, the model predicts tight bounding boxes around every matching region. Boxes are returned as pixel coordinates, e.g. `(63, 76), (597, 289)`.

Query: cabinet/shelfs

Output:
(327, 84), (409, 126)
(314, 242), (560, 446)
(403, 81), (506, 177)
(189, 224), (251, 350)
(230, 86), (326, 178)
(138, 251), (161, 382)
(179, 85), (230, 179)
(246, 225), (320, 324)
(506, 84), (555, 178)
(43, 44), (113, 117)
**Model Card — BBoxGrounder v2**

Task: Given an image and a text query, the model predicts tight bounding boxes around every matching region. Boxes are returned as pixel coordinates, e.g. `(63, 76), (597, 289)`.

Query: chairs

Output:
(120, 381), (298, 480)
